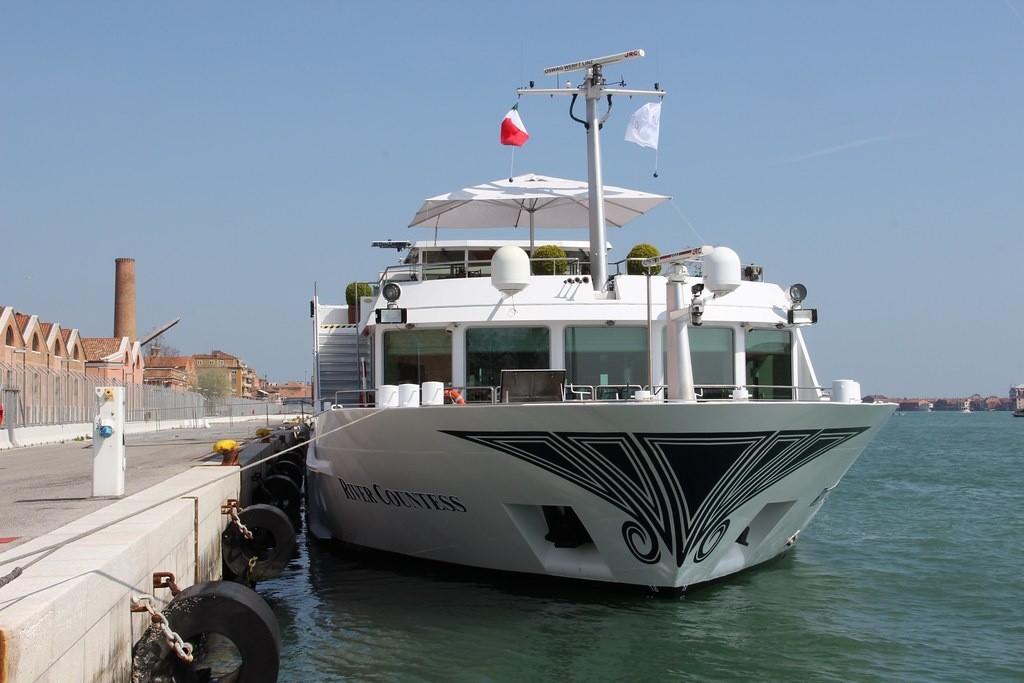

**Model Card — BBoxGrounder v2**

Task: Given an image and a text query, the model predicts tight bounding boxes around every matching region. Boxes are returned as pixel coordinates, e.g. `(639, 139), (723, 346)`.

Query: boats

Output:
(305, 49), (899, 589)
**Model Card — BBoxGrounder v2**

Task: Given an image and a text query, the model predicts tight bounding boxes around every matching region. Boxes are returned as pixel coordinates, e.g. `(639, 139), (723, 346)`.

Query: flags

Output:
(623, 101), (661, 151)
(500, 102), (530, 150)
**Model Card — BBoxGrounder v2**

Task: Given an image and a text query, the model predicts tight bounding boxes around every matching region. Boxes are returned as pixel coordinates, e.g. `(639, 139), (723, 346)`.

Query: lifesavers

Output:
(444, 389), (465, 405)
